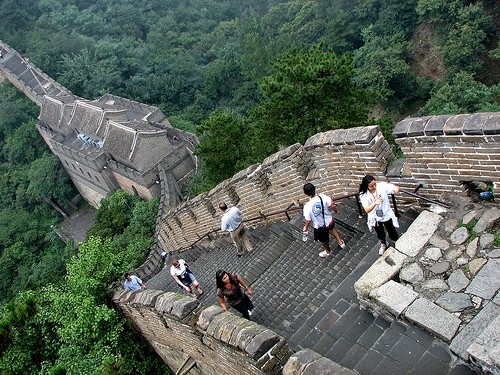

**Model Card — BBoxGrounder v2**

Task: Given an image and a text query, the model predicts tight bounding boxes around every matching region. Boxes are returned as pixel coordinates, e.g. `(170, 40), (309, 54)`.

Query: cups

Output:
(303, 232), (308, 241)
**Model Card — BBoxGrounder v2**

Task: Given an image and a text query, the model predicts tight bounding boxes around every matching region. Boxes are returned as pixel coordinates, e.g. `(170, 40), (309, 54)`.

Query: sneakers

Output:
(379, 241), (389, 255)
(338, 240), (345, 248)
(319, 250), (332, 257)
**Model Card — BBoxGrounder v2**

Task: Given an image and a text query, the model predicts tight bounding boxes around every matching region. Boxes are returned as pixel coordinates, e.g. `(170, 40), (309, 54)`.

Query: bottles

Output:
(377, 195), (383, 209)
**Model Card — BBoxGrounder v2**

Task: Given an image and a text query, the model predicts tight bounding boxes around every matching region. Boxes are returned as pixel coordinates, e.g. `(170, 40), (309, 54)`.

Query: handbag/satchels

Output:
(314, 227), (326, 242)
(240, 229), (245, 236)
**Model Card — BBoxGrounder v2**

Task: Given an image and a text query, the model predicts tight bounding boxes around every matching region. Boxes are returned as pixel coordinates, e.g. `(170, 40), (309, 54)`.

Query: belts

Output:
(231, 223), (241, 232)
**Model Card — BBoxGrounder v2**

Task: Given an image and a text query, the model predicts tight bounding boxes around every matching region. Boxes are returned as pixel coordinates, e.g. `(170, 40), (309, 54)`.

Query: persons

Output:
(359, 175), (400, 256)
(302, 183), (346, 257)
(219, 202), (253, 255)
(216, 270), (254, 321)
(170, 259), (204, 298)
(124, 273), (146, 292)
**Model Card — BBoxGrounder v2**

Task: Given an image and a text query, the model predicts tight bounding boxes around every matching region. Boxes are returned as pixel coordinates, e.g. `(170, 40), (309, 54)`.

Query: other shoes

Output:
(237, 254), (242, 256)
